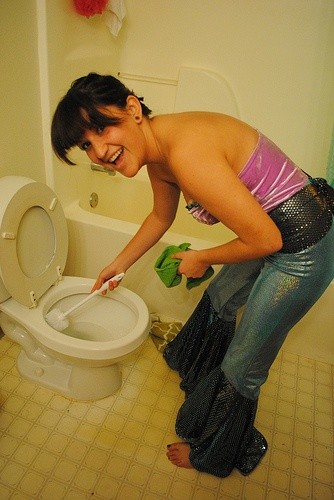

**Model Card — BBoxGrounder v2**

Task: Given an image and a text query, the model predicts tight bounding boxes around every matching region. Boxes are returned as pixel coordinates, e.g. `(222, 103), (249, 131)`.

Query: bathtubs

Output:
(52, 164), (334, 357)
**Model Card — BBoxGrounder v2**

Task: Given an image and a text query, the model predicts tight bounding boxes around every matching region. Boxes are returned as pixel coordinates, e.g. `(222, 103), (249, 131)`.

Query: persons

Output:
(50, 72), (334, 478)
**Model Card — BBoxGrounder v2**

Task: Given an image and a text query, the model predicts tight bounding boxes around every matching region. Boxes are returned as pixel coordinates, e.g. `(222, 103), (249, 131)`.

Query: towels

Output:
(153, 243), (215, 292)
(102, 0), (128, 39)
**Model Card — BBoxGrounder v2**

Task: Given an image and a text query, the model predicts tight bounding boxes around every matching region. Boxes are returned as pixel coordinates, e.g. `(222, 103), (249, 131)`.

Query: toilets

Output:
(1, 176), (150, 402)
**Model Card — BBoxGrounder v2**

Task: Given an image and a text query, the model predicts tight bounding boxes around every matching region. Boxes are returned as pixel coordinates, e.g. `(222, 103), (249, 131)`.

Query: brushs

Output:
(44, 272), (126, 332)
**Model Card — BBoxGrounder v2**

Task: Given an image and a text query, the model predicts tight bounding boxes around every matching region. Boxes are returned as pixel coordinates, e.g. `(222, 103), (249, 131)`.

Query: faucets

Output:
(90, 163), (116, 176)
